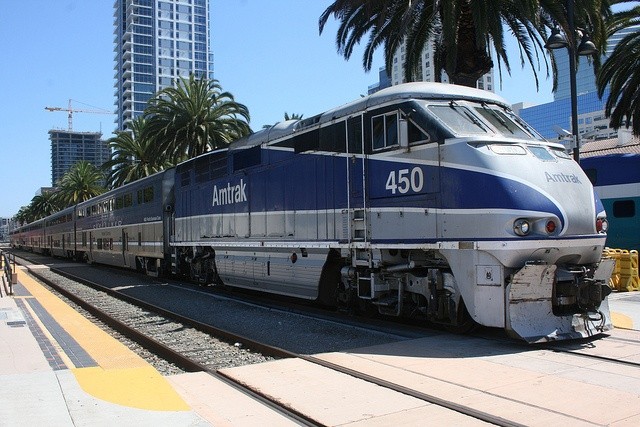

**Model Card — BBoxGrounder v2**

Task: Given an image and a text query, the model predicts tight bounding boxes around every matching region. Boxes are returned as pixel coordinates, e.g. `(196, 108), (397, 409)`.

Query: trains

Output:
(9, 81), (616, 345)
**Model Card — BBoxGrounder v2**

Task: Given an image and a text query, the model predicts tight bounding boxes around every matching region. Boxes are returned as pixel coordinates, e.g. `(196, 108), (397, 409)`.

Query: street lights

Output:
(544, 1), (597, 164)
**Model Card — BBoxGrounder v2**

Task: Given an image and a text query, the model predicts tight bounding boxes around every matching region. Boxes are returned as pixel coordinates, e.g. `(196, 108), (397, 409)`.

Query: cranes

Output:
(45, 98), (114, 130)
